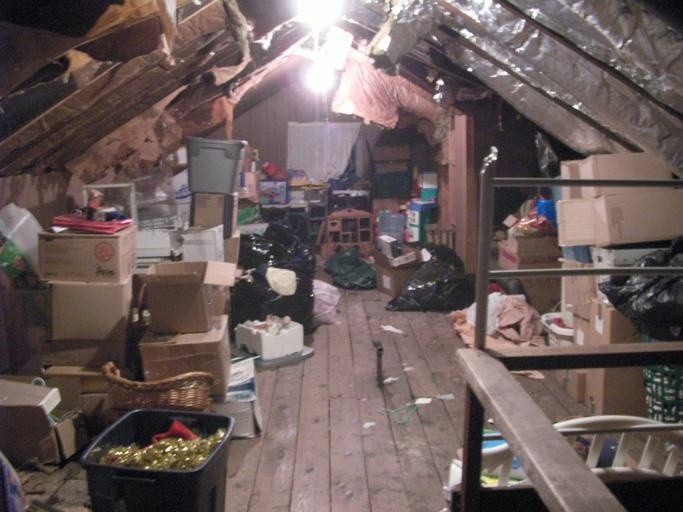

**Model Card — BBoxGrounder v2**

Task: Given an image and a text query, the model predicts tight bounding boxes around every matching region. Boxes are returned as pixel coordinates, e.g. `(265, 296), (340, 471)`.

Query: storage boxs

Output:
(0, 135), (304, 512)
(363, 136), (440, 299)
(499, 153), (683, 415)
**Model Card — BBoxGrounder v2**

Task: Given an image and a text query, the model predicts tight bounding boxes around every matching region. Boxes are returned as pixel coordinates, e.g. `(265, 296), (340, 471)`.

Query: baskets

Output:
(101, 359), (217, 412)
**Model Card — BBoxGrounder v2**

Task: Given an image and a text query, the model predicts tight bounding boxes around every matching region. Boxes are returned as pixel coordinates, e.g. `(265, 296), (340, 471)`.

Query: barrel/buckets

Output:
(0, 203), (48, 291)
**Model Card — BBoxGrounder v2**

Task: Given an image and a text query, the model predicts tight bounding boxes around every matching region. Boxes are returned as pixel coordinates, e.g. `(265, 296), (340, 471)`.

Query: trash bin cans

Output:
(81, 408), (236, 512)
